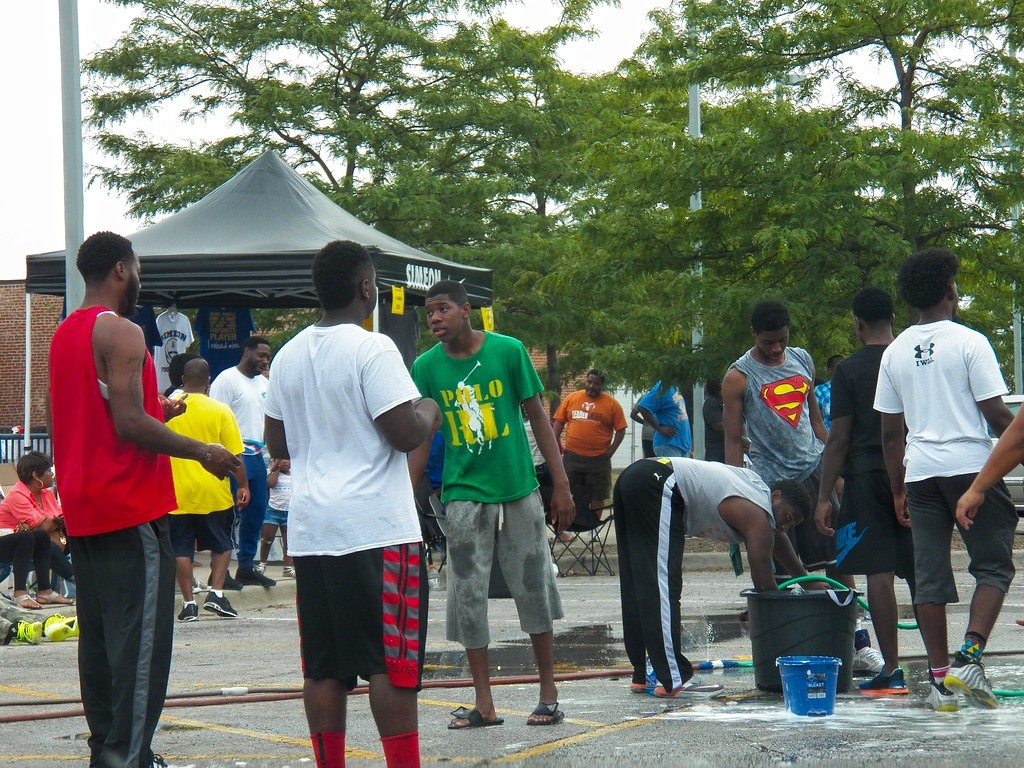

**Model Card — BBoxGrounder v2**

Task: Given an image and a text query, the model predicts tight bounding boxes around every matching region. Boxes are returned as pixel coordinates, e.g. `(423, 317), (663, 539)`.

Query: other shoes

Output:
(256, 562), (267, 576)
(282, 570), (296, 578)
(588, 527), (601, 542)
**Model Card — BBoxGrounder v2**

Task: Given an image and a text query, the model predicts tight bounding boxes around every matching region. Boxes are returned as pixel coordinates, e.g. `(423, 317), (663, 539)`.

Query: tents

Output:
(24, 149), (497, 459)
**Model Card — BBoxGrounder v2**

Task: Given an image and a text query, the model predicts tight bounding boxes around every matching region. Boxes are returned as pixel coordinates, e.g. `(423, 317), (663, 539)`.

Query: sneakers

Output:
(654, 680), (725, 699)
(208, 570), (243, 590)
(925, 681), (959, 712)
(203, 592), (237, 617)
(177, 604), (199, 622)
(236, 567), (276, 587)
(944, 651), (999, 710)
(192, 578), (212, 592)
(175, 587), (202, 594)
(852, 647), (885, 677)
(45, 612), (80, 642)
(859, 665), (908, 694)
(630, 681), (663, 693)
(12, 620), (42, 645)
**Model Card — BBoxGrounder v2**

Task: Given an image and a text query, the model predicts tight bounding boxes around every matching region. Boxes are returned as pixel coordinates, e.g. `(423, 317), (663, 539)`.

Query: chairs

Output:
(536, 472), (615, 576)
(414, 497), (447, 573)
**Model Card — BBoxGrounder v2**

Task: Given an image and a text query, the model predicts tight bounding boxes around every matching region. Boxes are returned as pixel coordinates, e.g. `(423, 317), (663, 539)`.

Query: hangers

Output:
(159, 289), (177, 317)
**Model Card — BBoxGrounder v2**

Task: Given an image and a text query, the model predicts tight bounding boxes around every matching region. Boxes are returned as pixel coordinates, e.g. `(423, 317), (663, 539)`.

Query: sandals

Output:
(11, 594), (42, 609)
(36, 591), (74, 604)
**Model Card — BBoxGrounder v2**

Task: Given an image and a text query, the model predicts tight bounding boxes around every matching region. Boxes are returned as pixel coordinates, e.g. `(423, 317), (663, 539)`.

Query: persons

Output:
(0, 451), (75, 585)
(704, 353), (852, 507)
(44, 231), (242, 768)
(422, 403), (556, 503)
(722, 300), (887, 680)
(955, 400), (1024, 531)
(814, 285), (936, 694)
(164, 350), (250, 623)
(548, 369), (627, 545)
(630, 379), (692, 458)
(872, 249), (1024, 711)
(614, 456), (813, 700)
(0, 530), (78, 647)
(403, 280), (576, 728)
(206, 337), (269, 589)
(264, 240), (442, 768)
(254, 458), (297, 579)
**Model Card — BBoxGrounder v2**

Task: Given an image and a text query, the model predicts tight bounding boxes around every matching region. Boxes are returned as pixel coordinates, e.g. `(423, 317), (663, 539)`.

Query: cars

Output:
(988, 394), (1024, 513)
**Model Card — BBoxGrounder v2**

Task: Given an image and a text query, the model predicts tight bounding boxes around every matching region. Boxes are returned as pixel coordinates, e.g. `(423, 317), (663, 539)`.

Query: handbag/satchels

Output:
(49, 528), (67, 551)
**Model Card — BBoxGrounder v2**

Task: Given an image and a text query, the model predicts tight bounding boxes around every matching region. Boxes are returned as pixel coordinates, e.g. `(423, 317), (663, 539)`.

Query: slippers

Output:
(556, 534), (578, 542)
(527, 702), (565, 725)
(448, 706), (504, 729)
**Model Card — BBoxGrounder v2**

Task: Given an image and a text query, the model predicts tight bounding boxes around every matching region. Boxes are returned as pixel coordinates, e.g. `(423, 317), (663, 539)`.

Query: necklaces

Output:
(38, 503), (42, 508)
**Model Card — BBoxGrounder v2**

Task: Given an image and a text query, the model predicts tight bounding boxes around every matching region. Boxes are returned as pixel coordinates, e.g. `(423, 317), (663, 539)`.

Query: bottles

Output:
(427, 564), (441, 590)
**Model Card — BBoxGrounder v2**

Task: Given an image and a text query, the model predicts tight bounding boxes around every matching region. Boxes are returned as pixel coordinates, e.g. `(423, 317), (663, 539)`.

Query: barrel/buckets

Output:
(776, 655), (842, 717)
(739, 586), (865, 692)
